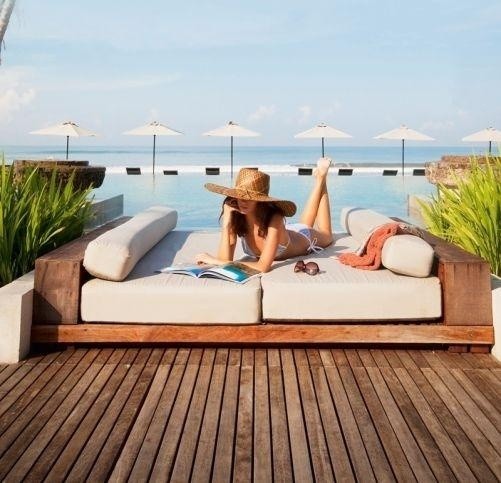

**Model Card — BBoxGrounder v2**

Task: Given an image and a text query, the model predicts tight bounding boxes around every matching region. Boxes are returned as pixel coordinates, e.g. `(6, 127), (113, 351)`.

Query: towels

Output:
(339, 221), (424, 270)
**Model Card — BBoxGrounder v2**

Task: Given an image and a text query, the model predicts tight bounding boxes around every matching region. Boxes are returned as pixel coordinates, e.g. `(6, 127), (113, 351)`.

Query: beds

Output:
(31, 204), (493, 353)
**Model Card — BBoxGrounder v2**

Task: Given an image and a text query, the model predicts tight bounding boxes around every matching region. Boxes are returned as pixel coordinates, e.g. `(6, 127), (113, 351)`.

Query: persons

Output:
(196, 155), (334, 273)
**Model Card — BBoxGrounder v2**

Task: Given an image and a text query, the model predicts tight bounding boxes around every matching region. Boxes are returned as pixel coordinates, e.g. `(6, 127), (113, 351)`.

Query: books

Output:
(154, 261), (265, 284)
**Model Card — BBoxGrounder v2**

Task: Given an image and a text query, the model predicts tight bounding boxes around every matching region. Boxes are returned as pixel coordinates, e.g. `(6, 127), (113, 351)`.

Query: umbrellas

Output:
(27, 120), (99, 160)
(202, 120), (260, 175)
(463, 125), (501, 155)
(373, 122), (435, 174)
(295, 121), (352, 158)
(124, 121), (183, 172)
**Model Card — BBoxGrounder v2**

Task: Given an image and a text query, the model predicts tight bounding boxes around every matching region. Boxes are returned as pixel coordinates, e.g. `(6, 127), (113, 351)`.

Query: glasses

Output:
(293, 260), (320, 276)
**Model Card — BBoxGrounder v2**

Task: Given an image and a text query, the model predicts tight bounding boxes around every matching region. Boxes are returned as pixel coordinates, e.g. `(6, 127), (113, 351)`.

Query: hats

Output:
(202, 167), (296, 217)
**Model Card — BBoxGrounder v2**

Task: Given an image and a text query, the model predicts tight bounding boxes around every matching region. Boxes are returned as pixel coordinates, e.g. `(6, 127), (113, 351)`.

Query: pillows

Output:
(339, 206), (434, 277)
(84, 206), (177, 281)
